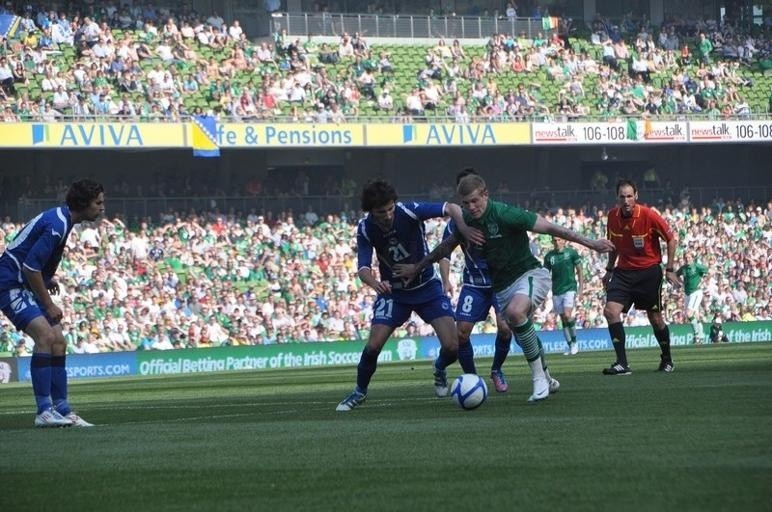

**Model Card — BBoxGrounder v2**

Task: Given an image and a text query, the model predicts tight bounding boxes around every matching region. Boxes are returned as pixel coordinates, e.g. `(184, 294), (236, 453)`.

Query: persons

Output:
(434, 166), (514, 397)
(602, 175), (683, 376)
(424, 216), (451, 253)
(335, 179), (487, 412)
(391, 310), (437, 337)
(2, 0), (396, 123)
(434, 244), (465, 310)
(674, 251), (714, 345)
(390, 173), (618, 404)
(1, 181), (69, 255)
(0, 312), (35, 357)
(543, 234), (583, 355)
(709, 316), (729, 343)
(496, 165), (772, 331)
(0, 178), (106, 427)
(403, 1), (771, 123)
(53, 177), (381, 355)
(470, 305), (497, 334)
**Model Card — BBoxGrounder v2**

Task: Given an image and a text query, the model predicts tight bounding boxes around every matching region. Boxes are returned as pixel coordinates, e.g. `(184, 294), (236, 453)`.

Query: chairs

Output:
(0, 17), (772, 124)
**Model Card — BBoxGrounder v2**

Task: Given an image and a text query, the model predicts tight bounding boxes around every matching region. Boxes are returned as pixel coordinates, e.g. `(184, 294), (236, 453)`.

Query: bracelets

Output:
(665, 267), (674, 272)
(606, 266), (614, 272)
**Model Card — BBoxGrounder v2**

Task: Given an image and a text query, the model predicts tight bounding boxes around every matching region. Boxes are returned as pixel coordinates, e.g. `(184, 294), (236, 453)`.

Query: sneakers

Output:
(564, 342), (579, 356)
(655, 362), (675, 374)
(490, 368), (508, 392)
(432, 359), (449, 397)
(335, 388), (368, 411)
(603, 362), (633, 376)
(33, 408), (94, 428)
(528, 377), (560, 402)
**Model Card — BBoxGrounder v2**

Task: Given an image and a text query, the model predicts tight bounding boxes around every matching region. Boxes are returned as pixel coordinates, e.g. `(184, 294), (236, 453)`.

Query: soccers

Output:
(451, 374), (488, 410)
